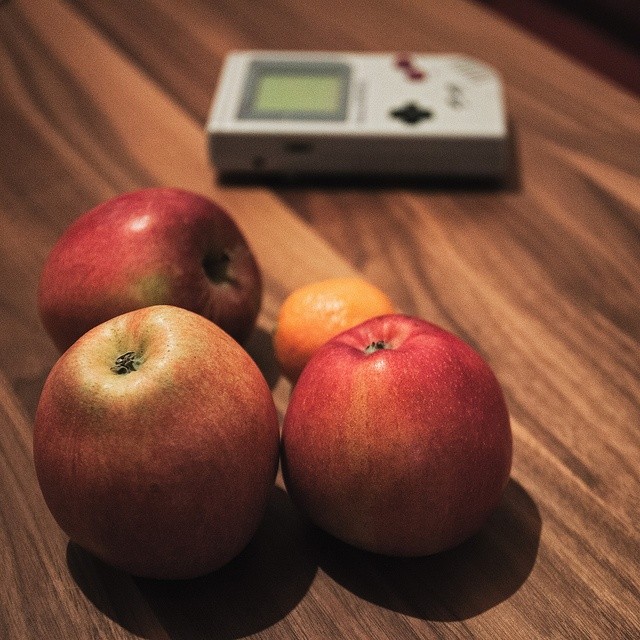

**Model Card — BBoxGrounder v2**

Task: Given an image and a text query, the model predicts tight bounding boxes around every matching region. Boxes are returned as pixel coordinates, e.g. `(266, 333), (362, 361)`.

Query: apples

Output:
(281, 313), (515, 556)
(35, 303), (285, 579)
(36, 190), (264, 352)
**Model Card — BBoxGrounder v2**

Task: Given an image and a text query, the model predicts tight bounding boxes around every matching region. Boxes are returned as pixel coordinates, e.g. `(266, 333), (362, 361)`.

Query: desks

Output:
(0, 0), (639, 639)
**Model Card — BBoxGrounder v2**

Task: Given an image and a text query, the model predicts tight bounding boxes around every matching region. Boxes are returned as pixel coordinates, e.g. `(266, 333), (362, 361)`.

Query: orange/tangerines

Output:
(278, 275), (393, 377)
